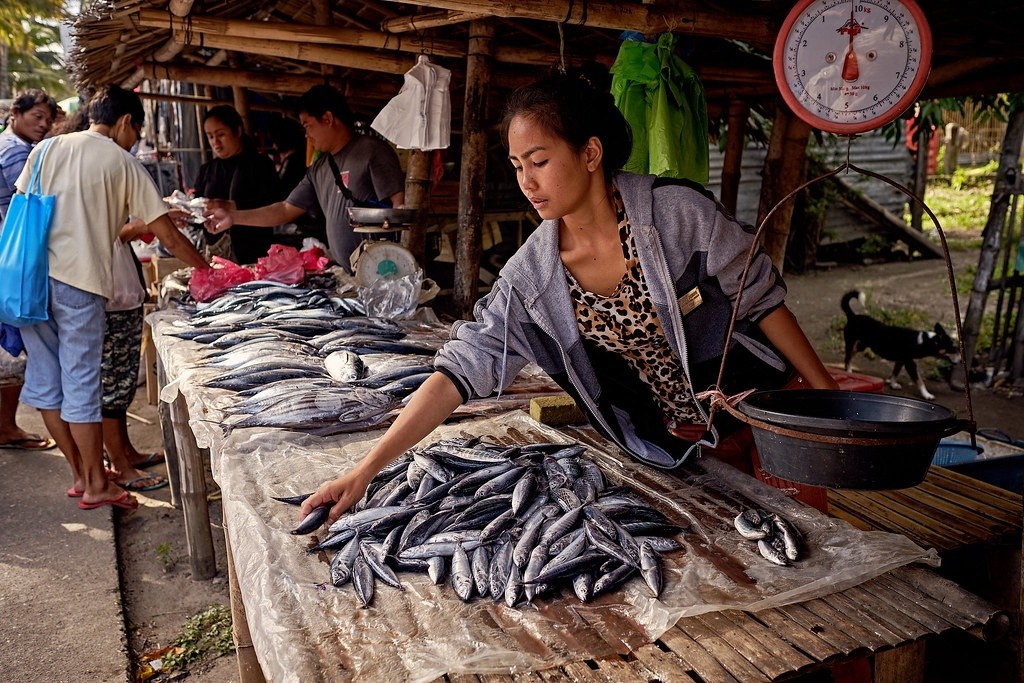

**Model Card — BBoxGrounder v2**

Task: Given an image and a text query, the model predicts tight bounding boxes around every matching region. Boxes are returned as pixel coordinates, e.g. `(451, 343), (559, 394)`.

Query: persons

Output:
(59, 99), (169, 494)
(297, 56), (840, 529)
(266, 115), (307, 194)
(202, 82), (408, 278)
(0, 86), (58, 452)
(10, 84), (214, 511)
(187, 103), (282, 264)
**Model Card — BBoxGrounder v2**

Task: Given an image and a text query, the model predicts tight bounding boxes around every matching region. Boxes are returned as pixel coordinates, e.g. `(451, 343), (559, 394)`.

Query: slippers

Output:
(0, 433), (57, 451)
(64, 470), (139, 511)
(114, 451), (169, 491)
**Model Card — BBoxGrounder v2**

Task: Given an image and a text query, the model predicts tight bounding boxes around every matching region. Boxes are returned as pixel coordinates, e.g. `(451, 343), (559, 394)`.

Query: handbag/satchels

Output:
(0, 139), (55, 329)
(106, 236), (145, 312)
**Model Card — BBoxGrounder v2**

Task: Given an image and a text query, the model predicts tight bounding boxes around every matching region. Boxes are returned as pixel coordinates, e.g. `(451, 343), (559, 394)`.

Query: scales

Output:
(346, 204), (421, 291)
(703, 5), (984, 495)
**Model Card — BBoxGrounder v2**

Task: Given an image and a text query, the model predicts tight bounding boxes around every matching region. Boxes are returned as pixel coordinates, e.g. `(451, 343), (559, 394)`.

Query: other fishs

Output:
(734, 506), (804, 566)
(162, 270), (439, 441)
(270, 432), (686, 609)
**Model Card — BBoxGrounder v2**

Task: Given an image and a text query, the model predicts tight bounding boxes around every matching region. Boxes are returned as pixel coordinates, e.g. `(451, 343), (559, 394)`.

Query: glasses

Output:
(130, 125), (143, 143)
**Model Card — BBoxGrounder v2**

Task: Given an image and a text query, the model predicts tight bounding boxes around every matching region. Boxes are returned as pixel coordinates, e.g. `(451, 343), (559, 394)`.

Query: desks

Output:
(149, 308), (1010, 683)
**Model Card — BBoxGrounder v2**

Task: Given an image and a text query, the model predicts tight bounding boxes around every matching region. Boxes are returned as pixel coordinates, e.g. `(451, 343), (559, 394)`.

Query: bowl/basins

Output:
(739, 389), (959, 491)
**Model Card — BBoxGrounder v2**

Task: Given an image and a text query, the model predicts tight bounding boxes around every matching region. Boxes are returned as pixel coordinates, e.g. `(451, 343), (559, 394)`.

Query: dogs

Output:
(840, 288), (963, 402)
